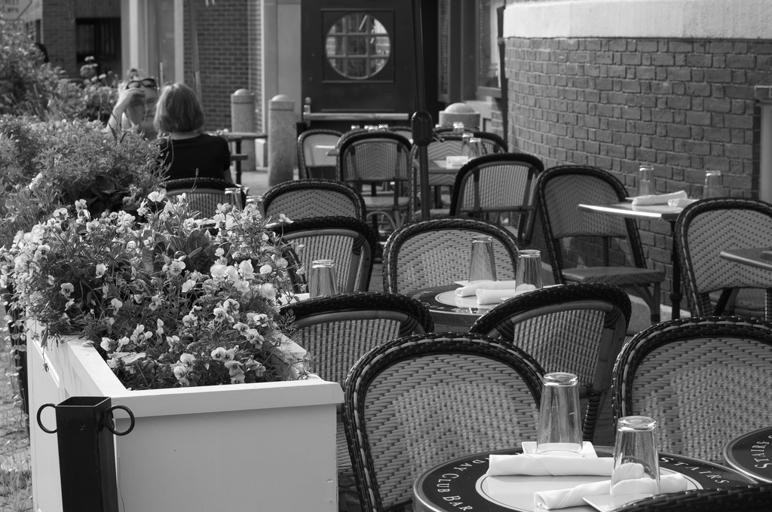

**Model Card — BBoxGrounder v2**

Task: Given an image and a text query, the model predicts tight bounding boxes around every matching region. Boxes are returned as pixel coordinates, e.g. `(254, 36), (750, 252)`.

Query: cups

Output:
(701, 168), (724, 200)
(466, 234), (497, 284)
(466, 137), (483, 160)
(307, 256), (338, 298)
(607, 414), (662, 507)
(511, 247), (543, 291)
(635, 162), (656, 197)
(535, 370), (584, 460)
(460, 132), (473, 155)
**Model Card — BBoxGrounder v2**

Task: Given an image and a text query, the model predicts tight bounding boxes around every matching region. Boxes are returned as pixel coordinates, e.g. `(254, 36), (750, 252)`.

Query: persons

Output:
(106, 76), (160, 140)
(150, 81), (232, 184)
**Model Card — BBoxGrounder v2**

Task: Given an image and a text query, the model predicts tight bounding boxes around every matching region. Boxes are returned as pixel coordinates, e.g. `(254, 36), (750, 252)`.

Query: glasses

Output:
(124, 78), (157, 90)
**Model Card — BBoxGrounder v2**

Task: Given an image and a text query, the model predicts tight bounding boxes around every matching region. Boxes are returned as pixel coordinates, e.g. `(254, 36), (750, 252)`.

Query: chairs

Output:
(328, 129), (412, 263)
(295, 128), (344, 183)
(344, 330), (554, 512)
(536, 162), (667, 326)
(252, 217), (375, 304)
(152, 179), (246, 227)
(273, 291), (435, 472)
(382, 217), (519, 301)
(447, 151), (546, 248)
(259, 179), (365, 231)
(610, 313), (772, 461)
(676, 196), (772, 320)
(410, 126), (506, 217)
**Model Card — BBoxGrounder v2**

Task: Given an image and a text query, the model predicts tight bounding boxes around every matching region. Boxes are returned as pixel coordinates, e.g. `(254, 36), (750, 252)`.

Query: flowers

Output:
(1, 197), (307, 387)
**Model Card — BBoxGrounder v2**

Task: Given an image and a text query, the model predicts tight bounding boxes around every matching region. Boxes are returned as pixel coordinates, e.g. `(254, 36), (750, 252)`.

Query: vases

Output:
(19, 315), (344, 512)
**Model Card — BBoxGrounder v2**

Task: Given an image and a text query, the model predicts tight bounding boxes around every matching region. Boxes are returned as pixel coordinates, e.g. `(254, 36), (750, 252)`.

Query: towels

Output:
(486, 454), (618, 476)
(455, 280), (523, 298)
(630, 190), (687, 206)
(666, 198), (701, 208)
(535, 473), (687, 510)
(475, 284), (565, 304)
